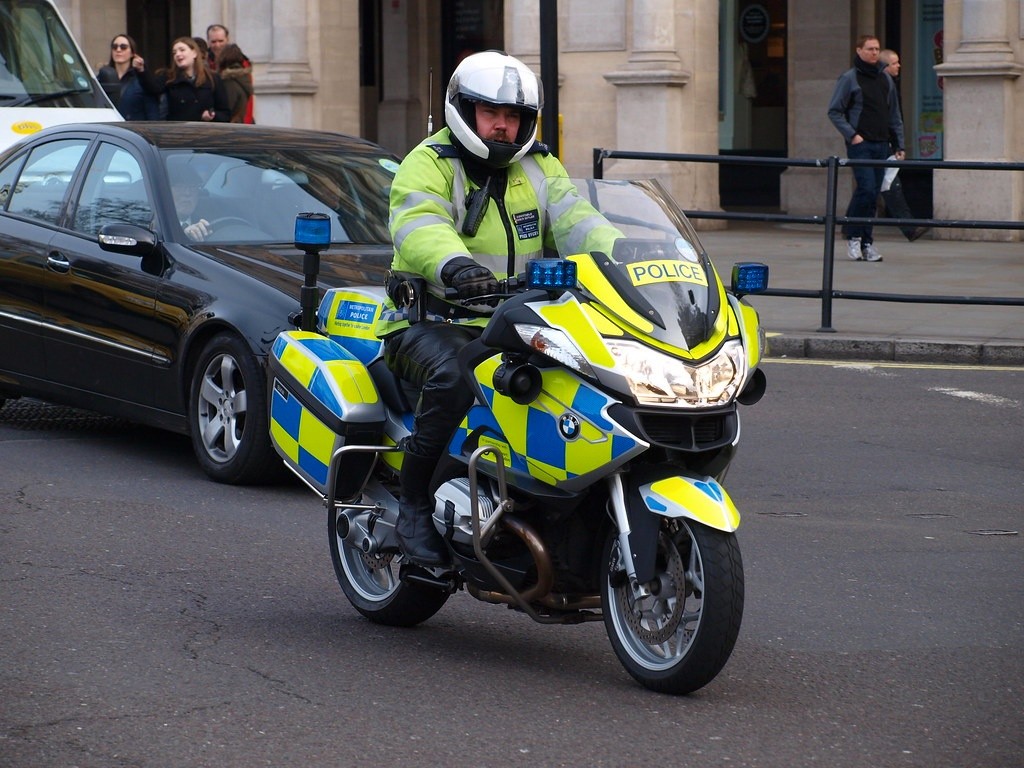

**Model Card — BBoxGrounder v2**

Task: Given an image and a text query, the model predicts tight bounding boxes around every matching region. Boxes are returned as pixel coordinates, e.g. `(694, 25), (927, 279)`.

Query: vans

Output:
(1, 0), (146, 180)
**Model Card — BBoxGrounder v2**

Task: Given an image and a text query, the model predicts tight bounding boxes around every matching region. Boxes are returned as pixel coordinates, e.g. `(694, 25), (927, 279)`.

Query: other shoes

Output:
(909, 226), (929, 241)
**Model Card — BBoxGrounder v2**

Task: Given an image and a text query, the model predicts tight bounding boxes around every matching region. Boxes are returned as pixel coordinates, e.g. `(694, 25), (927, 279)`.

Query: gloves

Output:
(441, 256), (502, 308)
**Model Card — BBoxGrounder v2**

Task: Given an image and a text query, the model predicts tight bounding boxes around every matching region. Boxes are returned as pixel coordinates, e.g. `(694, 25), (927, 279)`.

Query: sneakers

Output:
(862, 244), (883, 261)
(845, 237), (862, 260)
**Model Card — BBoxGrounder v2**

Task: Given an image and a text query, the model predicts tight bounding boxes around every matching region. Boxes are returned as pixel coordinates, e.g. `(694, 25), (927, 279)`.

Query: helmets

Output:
(445, 49), (544, 168)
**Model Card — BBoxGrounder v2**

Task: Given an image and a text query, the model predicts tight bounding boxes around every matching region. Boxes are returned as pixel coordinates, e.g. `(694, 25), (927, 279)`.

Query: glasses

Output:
(111, 44), (130, 50)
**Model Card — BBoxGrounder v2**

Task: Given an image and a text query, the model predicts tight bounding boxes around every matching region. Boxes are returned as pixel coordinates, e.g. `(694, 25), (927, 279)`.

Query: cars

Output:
(0, 120), (404, 484)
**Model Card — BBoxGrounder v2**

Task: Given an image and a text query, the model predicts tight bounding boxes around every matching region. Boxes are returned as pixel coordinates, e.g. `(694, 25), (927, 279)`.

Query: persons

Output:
(206, 22), (253, 126)
(132, 38), (232, 122)
(877, 49), (930, 242)
(97, 34), (161, 122)
(827, 36), (906, 261)
(151, 161), (215, 242)
(375, 48), (638, 568)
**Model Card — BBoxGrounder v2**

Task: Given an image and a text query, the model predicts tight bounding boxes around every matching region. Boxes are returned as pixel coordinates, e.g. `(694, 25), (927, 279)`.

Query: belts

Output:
(426, 294), (494, 319)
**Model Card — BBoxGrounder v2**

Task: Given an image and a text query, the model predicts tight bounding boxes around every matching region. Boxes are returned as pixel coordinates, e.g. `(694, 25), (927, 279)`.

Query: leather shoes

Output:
(394, 514), (455, 567)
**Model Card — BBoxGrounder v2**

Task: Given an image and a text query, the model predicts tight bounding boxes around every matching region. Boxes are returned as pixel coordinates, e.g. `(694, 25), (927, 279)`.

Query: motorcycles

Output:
(262, 177), (770, 696)
